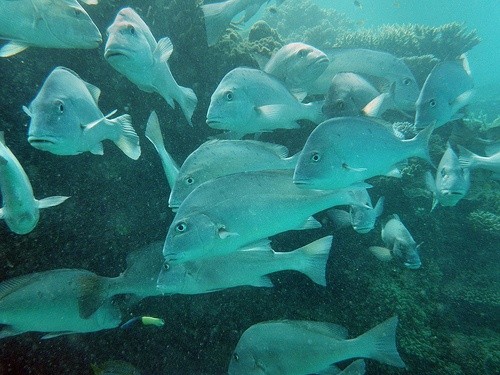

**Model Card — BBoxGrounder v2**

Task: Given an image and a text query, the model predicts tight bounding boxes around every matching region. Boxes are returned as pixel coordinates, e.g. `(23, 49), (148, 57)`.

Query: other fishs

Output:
(0, 0), (500, 375)
